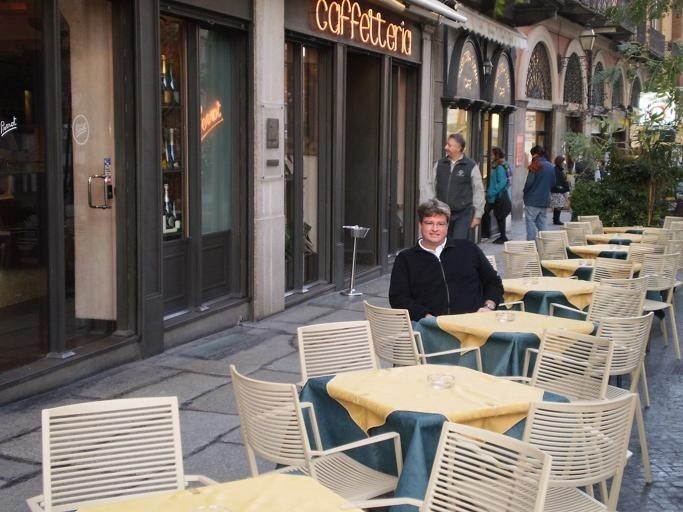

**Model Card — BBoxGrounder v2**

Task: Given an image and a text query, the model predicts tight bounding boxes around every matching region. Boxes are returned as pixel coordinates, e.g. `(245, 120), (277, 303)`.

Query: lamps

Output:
(482, 57), (494, 76)
(580, 18), (598, 55)
(561, 58), (569, 72)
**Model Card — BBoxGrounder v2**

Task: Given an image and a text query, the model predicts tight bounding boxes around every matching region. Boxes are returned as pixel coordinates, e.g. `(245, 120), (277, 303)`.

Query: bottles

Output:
(161, 54), (180, 229)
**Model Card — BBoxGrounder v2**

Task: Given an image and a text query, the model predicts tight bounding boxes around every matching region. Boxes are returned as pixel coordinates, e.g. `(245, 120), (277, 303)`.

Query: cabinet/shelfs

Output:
(159, 103), (183, 240)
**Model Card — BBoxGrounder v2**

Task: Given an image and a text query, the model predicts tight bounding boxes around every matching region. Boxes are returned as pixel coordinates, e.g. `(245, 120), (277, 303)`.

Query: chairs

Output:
(297, 216), (683, 511)
(230, 363), (404, 501)
(28, 395), (222, 512)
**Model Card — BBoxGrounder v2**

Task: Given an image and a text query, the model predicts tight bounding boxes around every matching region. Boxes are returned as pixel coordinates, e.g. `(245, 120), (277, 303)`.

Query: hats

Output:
(555, 156), (564, 165)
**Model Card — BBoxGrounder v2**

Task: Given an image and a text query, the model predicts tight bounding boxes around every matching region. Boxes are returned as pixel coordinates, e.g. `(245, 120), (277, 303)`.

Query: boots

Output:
(553, 210), (564, 225)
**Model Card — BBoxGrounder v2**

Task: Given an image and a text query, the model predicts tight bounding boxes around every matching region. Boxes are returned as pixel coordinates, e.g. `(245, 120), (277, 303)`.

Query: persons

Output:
(551, 156), (569, 224)
(390, 198), (505, 331)
(523, 145), (556, 240)
(481, 147), (512, 244)
(571, 159), (607, 187)
(432, 133), (486, 240)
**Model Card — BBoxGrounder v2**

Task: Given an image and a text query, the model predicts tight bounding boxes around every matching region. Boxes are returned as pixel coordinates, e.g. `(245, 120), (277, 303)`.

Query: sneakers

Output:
(493, 236), (507, 244)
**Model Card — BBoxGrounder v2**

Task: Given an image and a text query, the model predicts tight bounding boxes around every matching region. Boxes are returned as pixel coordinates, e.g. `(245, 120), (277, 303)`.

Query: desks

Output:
(79, 472), (362, 511)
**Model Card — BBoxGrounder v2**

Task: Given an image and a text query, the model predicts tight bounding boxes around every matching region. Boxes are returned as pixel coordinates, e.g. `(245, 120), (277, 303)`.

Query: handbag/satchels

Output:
(494, 190), (511, 219)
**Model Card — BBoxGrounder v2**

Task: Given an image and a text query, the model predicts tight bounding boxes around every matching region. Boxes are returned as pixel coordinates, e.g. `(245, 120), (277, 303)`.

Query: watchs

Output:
(484, 303), (496, 311)
